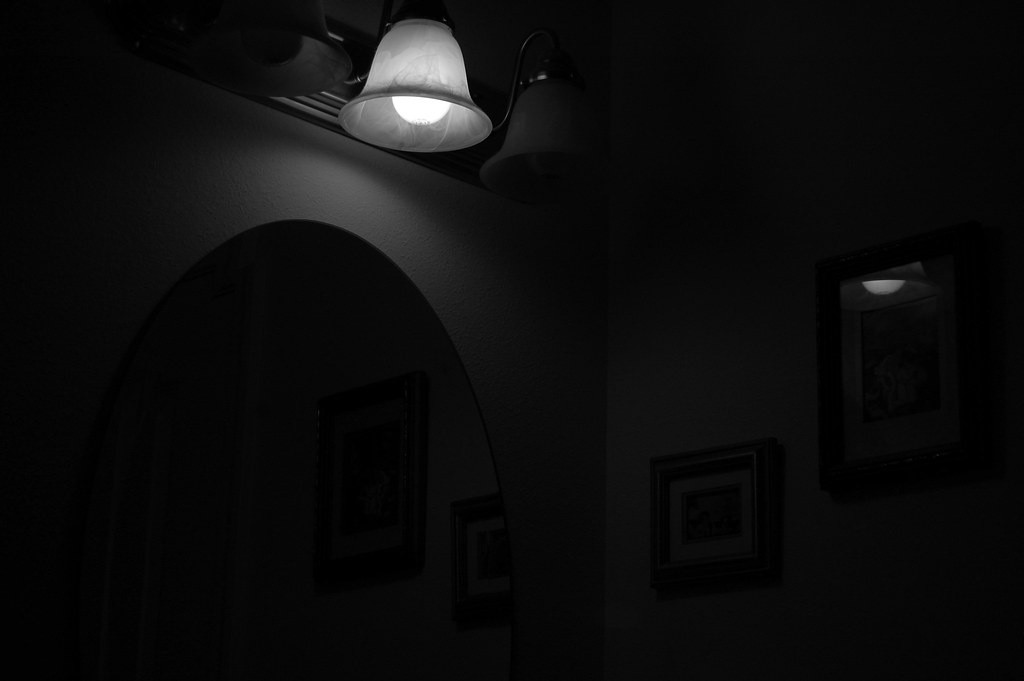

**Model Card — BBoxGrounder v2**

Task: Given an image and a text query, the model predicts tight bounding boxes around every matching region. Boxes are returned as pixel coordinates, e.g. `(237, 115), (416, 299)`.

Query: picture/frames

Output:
(814, 221), (983, 501)
(451, 491), (513, 624)
(312, 370), (429, 590)
(650, 437), (778, 594)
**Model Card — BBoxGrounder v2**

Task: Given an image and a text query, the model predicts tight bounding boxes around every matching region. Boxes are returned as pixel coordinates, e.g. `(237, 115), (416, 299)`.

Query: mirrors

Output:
(73, 220), (511, 681)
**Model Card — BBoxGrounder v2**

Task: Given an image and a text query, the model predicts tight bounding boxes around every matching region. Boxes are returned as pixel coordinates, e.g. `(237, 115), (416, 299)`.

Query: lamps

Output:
(841, 260), (935, 305)
(337, 21), (492, 154)
